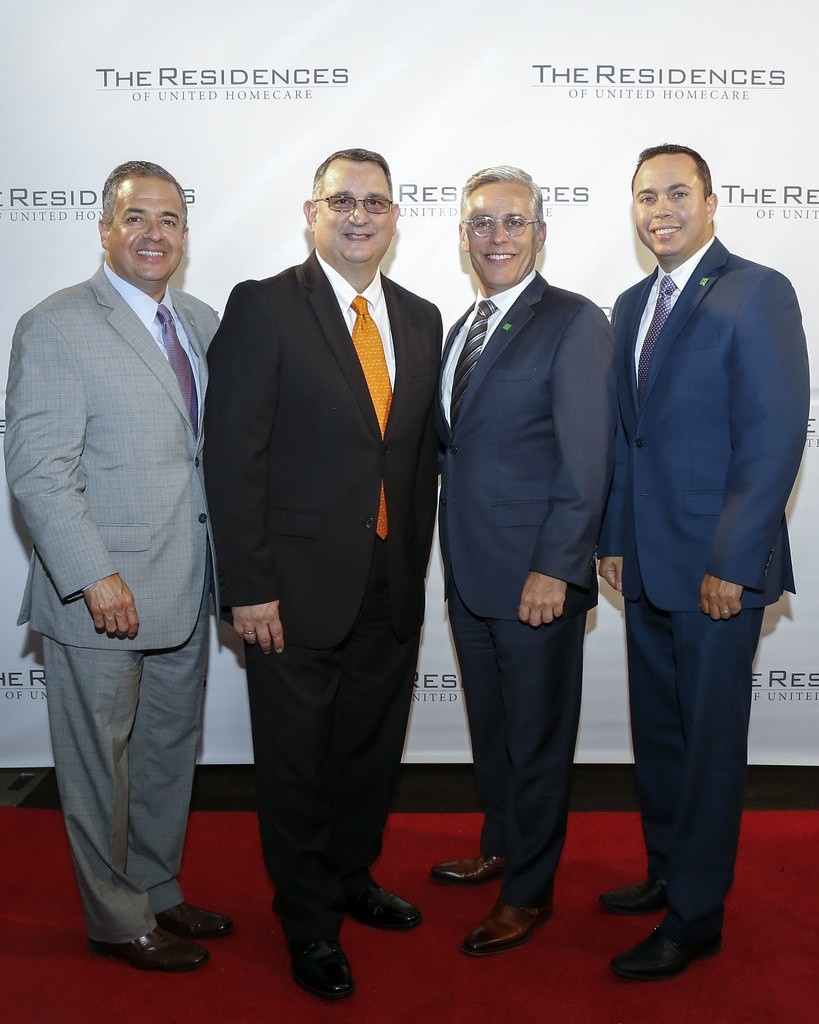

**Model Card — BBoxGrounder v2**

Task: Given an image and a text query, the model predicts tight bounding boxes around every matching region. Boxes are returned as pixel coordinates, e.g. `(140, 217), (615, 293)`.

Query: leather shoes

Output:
(430, 855), (506, 885)
(610, 925), (722, 979)
(347, 879), (421, 931)
(154, 902), (233, 940)
(291, 936), (354, 999)
(599, 883), (668, 914)
(91, 925), (210, 972)
(462, 899), (553, 956)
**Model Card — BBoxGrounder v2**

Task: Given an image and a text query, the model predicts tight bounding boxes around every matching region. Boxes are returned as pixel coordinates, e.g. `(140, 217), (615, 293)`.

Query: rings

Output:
(719, 609), (730, 615)
(242, 628), (256, 634)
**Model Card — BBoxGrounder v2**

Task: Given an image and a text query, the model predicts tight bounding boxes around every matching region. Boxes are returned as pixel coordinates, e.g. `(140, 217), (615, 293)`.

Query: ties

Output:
(351, 295), (388, 540)
(450, 300), (499, 430)
(637, 275), (678, 402)
(157, 304), (198, 439)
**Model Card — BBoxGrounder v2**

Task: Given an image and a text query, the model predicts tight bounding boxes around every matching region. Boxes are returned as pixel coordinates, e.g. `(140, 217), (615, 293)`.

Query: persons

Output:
(599, 142), (810, 980)
(202, 149), (443, 1000)
(431, 165), (615, 958)
(4, 160), (235, 973)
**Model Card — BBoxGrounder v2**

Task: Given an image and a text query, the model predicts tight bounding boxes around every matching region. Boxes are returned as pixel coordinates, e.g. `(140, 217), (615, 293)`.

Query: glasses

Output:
(313, 196), (393, 214)
(462, 215), (539, 237)
